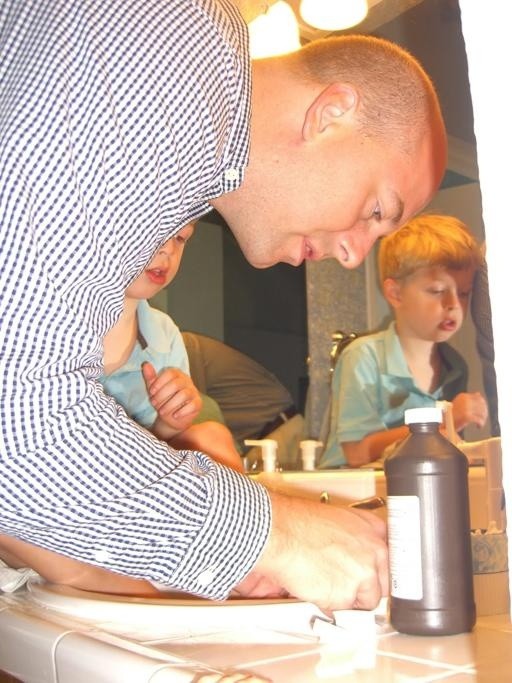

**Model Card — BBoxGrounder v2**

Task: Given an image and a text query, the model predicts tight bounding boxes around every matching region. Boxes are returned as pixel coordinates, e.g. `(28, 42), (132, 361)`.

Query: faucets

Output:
(432, 398), (502, 531)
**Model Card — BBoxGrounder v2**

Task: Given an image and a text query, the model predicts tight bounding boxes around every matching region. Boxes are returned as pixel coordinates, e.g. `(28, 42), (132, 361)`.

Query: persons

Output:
(94, 205), (248, 475)
(316, 209), (490, 469)
(181, 330), (306, 472)
(0, 1), (448, 618)
(470, 241), (501, 441)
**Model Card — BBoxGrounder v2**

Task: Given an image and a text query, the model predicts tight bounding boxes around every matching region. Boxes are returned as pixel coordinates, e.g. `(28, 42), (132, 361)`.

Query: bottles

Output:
(383, 408), (476, 636)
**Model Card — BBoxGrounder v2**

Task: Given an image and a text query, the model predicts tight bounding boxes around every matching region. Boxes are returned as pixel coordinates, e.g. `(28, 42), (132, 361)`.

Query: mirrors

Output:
(146, 0), (502, 475)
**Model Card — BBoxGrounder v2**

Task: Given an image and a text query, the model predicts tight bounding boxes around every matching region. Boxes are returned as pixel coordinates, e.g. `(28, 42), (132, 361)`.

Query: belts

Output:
(258, 405), (298, 439)
